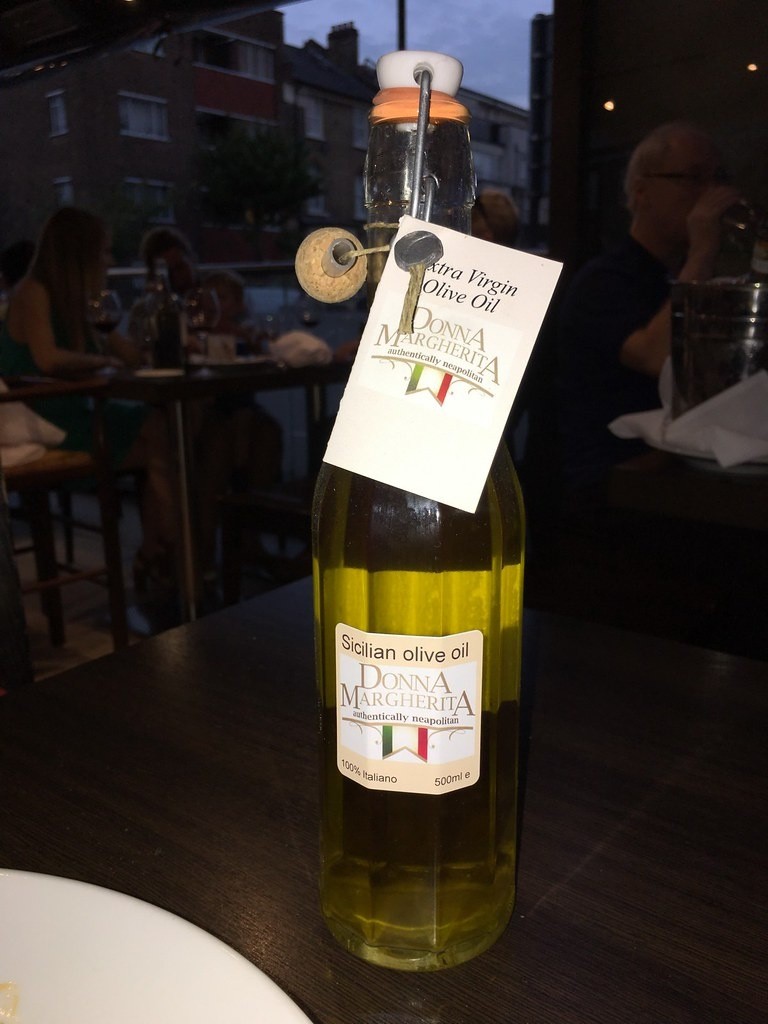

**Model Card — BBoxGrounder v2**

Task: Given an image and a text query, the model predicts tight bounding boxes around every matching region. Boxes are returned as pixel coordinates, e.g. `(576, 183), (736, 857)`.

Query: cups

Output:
(208, 334), (236, 364)
(297, 300), (322, 332)
(722, 204), (768, 238)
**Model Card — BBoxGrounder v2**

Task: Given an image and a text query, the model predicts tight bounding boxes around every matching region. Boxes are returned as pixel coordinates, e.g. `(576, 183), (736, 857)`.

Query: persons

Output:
(558, 122), (768, 658)
(0, 207), (285, 621)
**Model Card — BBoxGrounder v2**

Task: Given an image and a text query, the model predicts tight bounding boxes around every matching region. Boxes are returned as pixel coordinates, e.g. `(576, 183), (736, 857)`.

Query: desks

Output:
(51, 359), (351, 624)
(610, 451), (768, 530)
(2, 577), (768, 1024)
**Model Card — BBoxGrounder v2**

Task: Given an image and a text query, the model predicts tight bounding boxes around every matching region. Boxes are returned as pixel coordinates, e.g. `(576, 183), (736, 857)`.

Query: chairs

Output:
(0, 359), (353, 689)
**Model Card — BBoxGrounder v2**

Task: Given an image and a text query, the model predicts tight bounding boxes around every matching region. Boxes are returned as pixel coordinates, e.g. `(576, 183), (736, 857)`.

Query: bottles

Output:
(152, 274), (181, 368)
(750, 237), (768, 283)
(309, 49), (525, 969)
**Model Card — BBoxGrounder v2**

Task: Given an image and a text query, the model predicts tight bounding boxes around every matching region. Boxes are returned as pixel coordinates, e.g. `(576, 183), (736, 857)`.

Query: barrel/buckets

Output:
(670, 279), (768, 421)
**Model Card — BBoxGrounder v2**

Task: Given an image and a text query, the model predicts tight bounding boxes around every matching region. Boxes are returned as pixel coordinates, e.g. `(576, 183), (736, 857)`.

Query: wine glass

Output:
(191, 311), (212, 361)
(260, 309), (281, 359)
(91, 289), (124, 367)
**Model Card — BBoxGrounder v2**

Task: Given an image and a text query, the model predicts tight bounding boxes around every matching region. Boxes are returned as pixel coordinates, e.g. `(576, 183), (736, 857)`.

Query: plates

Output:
(0, 868), (314, 1024)
